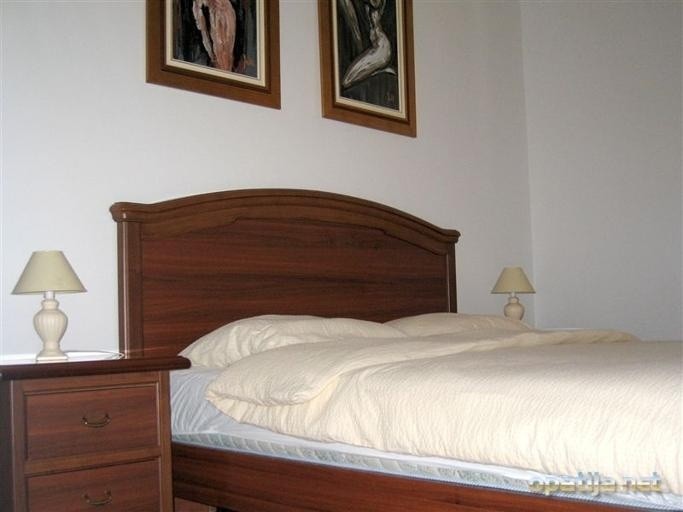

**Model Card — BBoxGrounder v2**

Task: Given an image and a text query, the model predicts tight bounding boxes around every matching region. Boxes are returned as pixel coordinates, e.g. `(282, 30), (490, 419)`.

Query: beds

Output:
(110, 191), (683, 512)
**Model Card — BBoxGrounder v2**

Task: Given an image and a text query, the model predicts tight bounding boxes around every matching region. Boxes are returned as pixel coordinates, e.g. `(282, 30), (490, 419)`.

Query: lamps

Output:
(11, 251), (88, 362)
(488, 264), (536, 322)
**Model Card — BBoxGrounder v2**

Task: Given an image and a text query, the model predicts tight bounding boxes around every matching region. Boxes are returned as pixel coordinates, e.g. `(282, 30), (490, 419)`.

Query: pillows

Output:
(381, 312), (535, 340)
(174, 307), (411, 367)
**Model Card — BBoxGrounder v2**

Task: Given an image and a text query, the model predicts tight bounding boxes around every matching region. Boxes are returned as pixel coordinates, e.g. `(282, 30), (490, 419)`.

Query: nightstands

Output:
(1, 355), (190, 511)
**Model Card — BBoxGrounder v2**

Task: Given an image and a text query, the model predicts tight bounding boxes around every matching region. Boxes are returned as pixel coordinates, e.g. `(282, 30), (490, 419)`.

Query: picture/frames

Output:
(317, 0), (416, 138)
(143, 0), (282, 110)
